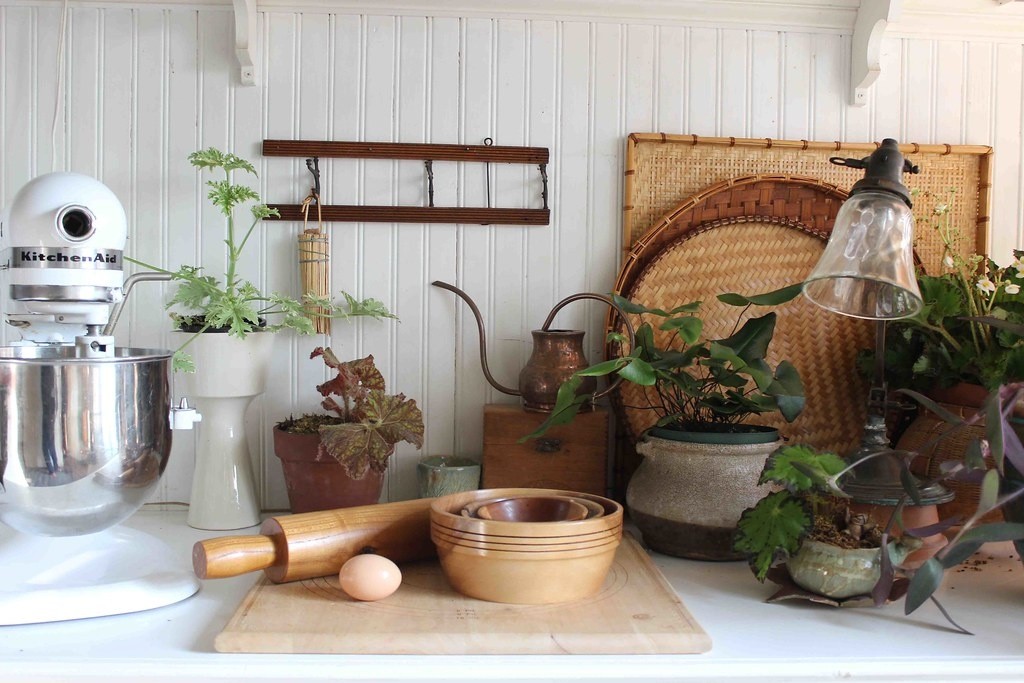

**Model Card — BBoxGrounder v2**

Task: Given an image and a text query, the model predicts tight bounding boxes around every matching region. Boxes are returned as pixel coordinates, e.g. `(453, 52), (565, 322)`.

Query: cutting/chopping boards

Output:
(214, 531), (711, 652)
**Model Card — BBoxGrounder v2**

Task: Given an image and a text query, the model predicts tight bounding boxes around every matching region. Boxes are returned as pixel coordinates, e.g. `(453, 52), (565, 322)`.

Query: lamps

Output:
(800, 139), (960, 504)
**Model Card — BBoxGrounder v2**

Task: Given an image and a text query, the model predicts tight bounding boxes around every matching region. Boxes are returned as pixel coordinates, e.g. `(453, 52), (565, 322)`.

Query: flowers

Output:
(852, 184), (1024, 397)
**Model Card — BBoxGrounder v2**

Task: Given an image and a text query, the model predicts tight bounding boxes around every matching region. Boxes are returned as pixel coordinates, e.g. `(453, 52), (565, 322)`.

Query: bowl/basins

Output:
(429, 488), (623, 602)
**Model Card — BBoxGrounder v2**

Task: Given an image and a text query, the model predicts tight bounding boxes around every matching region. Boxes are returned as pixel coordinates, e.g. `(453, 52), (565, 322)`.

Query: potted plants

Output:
(730, 441), (908, 606)
(523, 281), (826, 564)
(270, 348), (424, 514)
(124, 143), (400, 530)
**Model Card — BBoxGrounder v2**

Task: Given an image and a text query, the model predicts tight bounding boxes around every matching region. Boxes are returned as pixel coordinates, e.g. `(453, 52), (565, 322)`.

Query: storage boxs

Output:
(481, 402), (612, 498)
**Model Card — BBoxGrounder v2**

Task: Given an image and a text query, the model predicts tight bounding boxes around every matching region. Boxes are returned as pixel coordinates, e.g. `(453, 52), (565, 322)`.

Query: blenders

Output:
(0, 172), (200, 624)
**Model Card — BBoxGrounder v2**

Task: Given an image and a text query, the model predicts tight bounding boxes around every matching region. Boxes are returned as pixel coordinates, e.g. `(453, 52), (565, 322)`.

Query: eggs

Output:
(339, 554), (402, 601)
